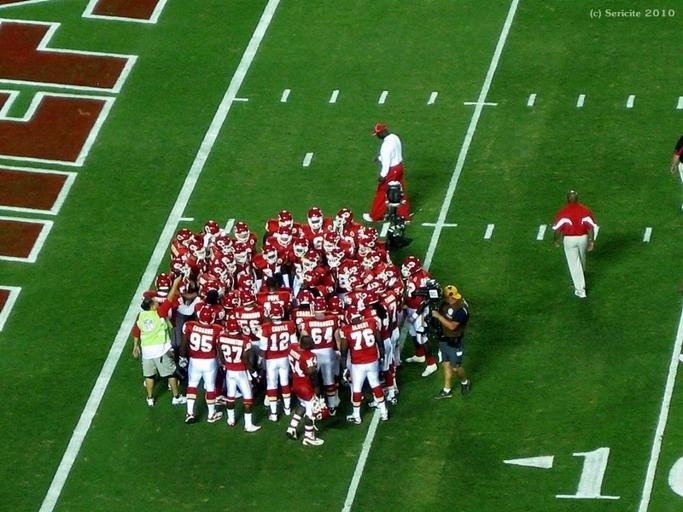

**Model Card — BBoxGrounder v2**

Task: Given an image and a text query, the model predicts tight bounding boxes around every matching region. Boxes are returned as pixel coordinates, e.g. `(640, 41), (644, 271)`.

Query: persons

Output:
(550, 188), (599, 299)
(667, 133), (681, 210)
(361, 120), (411, 222)
(130, 206), (471, 449)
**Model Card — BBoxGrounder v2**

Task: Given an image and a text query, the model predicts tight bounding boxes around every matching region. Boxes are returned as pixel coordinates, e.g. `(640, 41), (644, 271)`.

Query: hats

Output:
(442, 285), (463, 301)
(369, 123), (386, 137)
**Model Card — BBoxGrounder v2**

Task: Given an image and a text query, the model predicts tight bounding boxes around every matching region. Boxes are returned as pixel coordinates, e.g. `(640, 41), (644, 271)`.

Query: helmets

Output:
(143, 251), (290, 328)
(340, 227), (380, 255)
(203, 221), (218, 242)
(294, 256), (420, 323)
(336, 208), (353, 226)
(278, 210), (294, 229)
(276, 229), (293, 248)
(324, 232), (337, 252)
(217, 238), (235, 256)
(234, 222), (249, 242)
(294, 239), (308, 258)
(176, 229), (195, 248)
(306, 208), (323, 229)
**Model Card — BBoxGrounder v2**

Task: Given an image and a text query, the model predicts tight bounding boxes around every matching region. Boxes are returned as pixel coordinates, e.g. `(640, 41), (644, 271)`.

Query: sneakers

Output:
(301, 437), (326, 447)
(369, 373), (398, 421)
(166, 355), (188, 406)
(362, 213), (386, 223)
(328, 369), (362, 425)
(264, 394), (292, 420)
(286, 427), (300, 439)
(460, 379), (471, 396)
(184, 370), (262, 433)
(146, 398), (156, 409)
(405, 355), (427, 363)
(573, 290), (587, 297)
(420, 363), (438, 378)
(433, 388), (455, 400)
